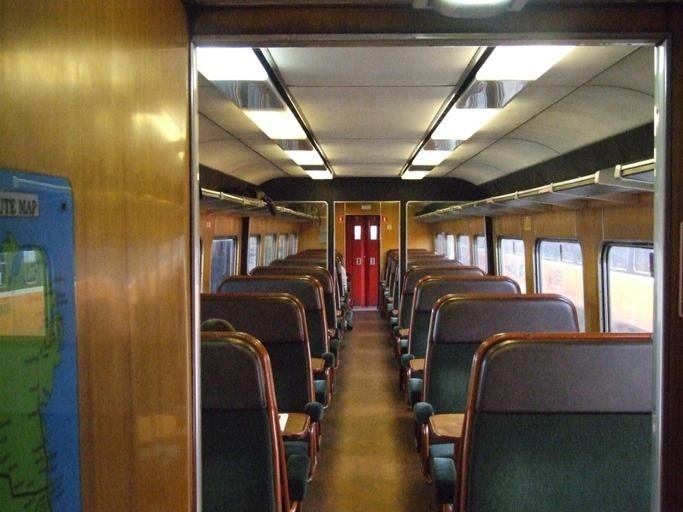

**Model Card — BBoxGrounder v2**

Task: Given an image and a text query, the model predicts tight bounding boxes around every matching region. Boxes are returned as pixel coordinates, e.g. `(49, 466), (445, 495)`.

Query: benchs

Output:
(430, 330), (654, 512)
(416, 292), (581, 483)
(200, 330), (315, 512)
(210, 247), (353, 408)
(377, 249), (523, 396)
(201, 290), (326, 453)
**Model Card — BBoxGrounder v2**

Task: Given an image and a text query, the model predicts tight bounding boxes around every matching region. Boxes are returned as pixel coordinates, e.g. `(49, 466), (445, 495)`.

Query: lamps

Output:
(400, 45), (573, 181)
(196, 46), (332, 180)
